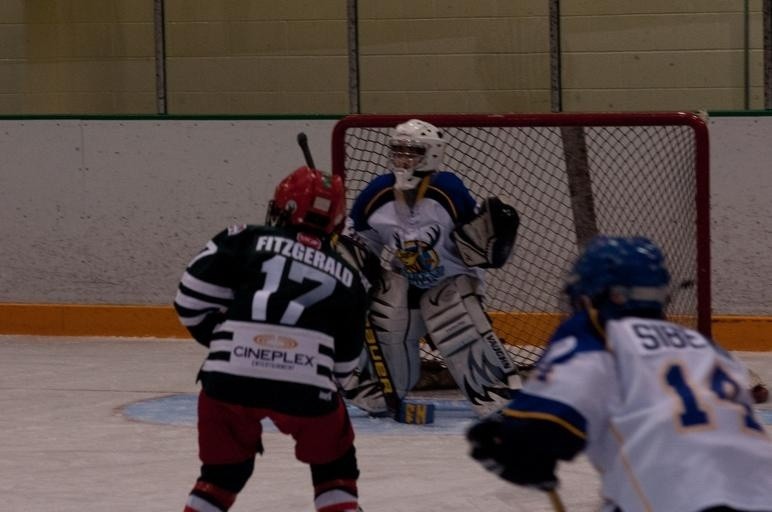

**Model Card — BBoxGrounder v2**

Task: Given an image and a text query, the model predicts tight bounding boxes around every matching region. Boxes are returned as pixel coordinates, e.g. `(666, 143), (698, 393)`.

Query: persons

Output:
(347, 119), (527, 419)
(172, 165), (371, 512)
(466, 232), (772, 512)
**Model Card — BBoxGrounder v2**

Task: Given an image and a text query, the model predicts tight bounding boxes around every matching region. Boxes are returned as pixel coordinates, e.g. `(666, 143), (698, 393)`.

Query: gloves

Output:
(468, 439), (559, 493)
(340, 370), (386, 414)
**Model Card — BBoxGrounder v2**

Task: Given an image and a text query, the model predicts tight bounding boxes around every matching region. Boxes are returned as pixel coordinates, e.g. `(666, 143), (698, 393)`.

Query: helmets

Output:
(275, 167), (348, 245)
(390, 118), (444, 189)
(558, 233), (672, 332)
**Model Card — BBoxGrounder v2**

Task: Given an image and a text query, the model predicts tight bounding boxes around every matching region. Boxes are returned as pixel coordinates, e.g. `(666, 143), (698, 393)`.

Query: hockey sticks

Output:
(297, 132), (435, 423)
